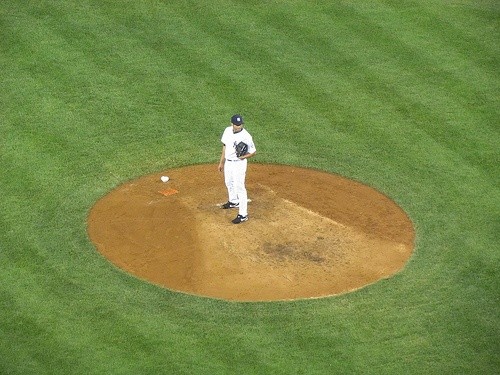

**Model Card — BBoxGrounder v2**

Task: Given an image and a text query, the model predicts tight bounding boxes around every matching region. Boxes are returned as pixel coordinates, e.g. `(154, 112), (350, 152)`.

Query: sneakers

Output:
(232, 215), (248, 224)
(223, 201), (239, 209)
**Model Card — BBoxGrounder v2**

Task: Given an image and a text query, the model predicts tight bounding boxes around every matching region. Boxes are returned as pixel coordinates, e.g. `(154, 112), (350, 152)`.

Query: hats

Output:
(231, 115), (244, 125)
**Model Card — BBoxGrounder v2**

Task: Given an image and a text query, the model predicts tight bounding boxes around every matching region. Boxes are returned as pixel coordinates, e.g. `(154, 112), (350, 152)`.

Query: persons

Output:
(218, 114), (256, 224)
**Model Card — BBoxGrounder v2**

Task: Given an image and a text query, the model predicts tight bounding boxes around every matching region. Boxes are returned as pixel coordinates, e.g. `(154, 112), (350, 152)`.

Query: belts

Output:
(227, 160), (232, 161)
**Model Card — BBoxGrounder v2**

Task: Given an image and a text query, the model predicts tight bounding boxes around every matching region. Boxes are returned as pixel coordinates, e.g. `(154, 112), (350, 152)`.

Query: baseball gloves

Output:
(235, 141), (248, 157)
